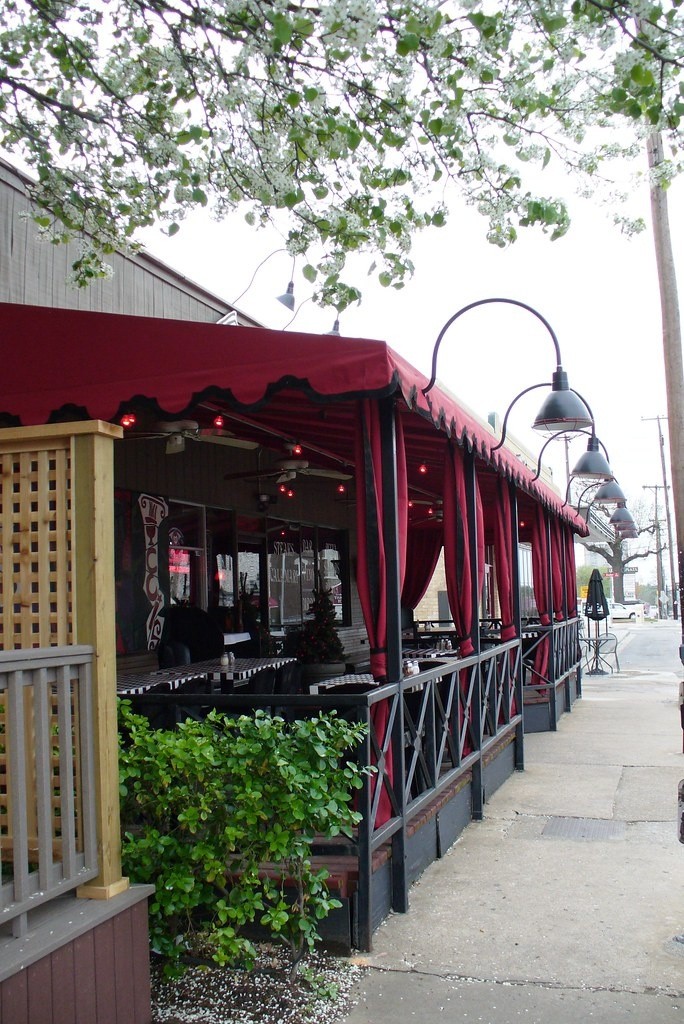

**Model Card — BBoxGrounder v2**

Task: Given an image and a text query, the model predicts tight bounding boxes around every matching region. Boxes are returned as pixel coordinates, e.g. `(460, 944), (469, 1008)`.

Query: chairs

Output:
(140, 678), (212, 695)
(248, 662), (306, 694)
(594, 633), (621, 672)
(577, 633), (591, 676)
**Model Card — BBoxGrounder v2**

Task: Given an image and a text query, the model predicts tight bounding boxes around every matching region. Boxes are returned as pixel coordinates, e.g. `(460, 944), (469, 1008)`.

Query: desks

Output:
(307, 632), (540, 694)
(577, 638), (616, 677)
(148, 657), (298, 695)
(50, 672), (207, 695)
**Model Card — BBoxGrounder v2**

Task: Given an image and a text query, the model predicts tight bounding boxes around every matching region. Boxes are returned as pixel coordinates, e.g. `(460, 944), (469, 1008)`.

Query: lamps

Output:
(232, 249), (296, 311)
(491, 382), (614, 480)
(422, 298), (594, 442)
(282, 297), (340, 336)
(531, 429), (638, 538)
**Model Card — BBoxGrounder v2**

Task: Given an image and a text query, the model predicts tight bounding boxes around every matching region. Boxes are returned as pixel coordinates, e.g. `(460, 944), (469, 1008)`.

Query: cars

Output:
(591, 603), (640, 620)
(641, 601), (651, 614)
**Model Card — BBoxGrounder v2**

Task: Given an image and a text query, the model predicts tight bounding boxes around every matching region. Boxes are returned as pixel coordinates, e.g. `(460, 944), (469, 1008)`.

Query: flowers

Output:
(294, 569), (344, 665)
(229, 572), (279, 656)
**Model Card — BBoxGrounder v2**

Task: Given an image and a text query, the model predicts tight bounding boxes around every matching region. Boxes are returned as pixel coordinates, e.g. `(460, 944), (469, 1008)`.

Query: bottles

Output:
(404, 660), (420, 674)
(425, 622), (432, 630)
(437, 639), (452, 652)
(416, 620), (419, 630)
(220, 652), (235, 667)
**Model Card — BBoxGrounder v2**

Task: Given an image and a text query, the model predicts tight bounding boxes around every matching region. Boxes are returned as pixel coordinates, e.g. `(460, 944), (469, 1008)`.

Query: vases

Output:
(301, 664), (346, 689)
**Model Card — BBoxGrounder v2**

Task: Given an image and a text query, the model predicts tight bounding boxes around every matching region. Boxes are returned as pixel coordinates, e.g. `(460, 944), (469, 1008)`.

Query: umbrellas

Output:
(585, 569), (609, 669)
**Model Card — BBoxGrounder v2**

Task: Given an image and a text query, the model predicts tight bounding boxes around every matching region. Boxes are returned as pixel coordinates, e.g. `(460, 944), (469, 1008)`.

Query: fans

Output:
(224, 442), (353, 483)
(116, 416), (259, 454)
(407, 498), (445, 526)
